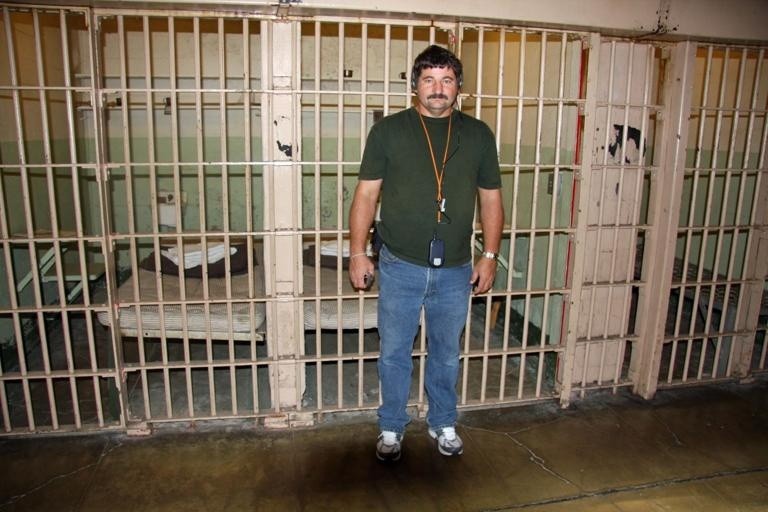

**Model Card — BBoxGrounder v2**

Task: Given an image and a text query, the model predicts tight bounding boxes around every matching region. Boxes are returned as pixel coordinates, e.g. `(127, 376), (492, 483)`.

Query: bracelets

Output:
(349, 252), (368, 261)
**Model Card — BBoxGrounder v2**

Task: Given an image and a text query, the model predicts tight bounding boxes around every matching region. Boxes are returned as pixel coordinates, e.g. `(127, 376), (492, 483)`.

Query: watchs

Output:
(480, 250), (498, 262)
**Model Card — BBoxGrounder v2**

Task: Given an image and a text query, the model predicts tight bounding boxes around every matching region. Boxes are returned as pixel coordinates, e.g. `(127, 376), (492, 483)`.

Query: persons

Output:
(348, 43), (505, 460)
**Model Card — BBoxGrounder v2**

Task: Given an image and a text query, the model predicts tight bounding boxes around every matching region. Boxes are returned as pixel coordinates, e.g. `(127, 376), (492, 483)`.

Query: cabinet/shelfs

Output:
(474, 223), (524, 279)
(9, 229), (106, 322)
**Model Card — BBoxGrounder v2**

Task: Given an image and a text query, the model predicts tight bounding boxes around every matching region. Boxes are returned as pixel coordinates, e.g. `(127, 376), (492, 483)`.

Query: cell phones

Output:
(429, 233), (446, 267)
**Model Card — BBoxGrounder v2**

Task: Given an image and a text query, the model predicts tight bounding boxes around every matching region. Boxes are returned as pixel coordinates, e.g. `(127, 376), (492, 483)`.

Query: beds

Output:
(98, 240), (266, 426)
(635, 243), (768, 350)
(304, 241), (422, 330)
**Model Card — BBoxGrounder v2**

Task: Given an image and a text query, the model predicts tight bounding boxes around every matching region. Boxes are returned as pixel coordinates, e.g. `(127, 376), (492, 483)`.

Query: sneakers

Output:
(375, 431), (404, 461)
(428, 427), (464, 456)
